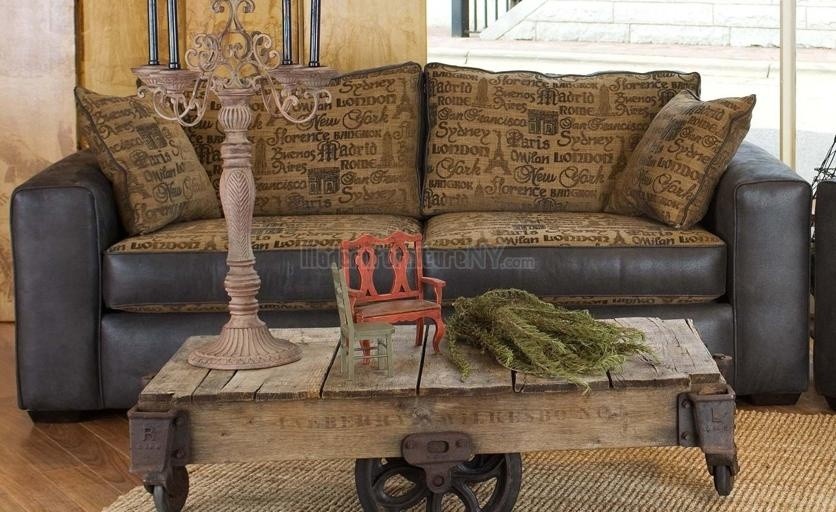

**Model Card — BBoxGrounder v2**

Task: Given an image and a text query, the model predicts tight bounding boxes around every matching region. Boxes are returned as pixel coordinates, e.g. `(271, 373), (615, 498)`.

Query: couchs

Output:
(10, 143), (813, 422)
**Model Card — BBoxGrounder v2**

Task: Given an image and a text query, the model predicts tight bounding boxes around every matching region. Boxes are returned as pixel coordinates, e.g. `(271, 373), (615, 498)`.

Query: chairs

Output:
(331, 230), (446, 380)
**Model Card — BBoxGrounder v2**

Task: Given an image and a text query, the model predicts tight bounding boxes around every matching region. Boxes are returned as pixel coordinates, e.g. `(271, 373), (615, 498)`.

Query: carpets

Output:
(98, 409), (834, 512)
